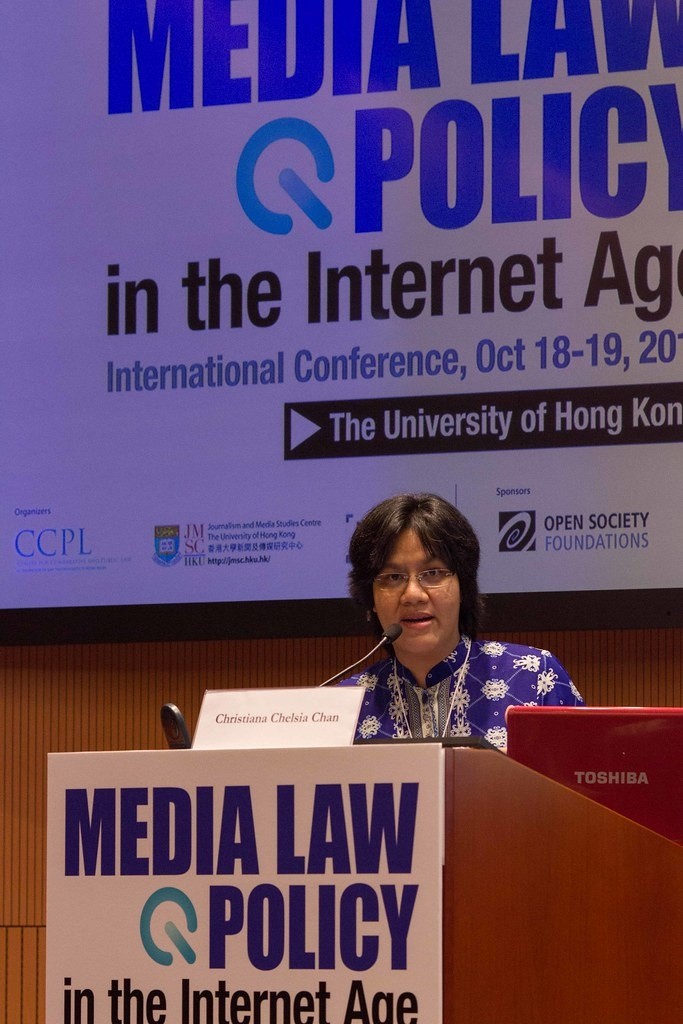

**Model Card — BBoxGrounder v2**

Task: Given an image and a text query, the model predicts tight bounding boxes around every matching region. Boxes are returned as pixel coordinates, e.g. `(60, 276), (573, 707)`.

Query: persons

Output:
(329, 491), (584, 754)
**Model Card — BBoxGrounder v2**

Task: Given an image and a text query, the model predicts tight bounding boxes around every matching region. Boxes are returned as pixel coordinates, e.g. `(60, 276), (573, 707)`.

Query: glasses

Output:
(373, 569), (456, 591)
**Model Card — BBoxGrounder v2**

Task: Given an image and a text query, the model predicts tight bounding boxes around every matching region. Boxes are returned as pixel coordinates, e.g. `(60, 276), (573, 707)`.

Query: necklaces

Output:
(394, 632), (471, 739)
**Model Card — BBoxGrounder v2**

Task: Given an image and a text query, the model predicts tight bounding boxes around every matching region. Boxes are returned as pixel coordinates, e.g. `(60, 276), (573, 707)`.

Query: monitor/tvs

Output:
(505, 704), (683, 849)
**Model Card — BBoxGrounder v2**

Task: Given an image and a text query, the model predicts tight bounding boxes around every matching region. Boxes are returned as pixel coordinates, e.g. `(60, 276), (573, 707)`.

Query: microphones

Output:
(319, 623), (402, 686)
